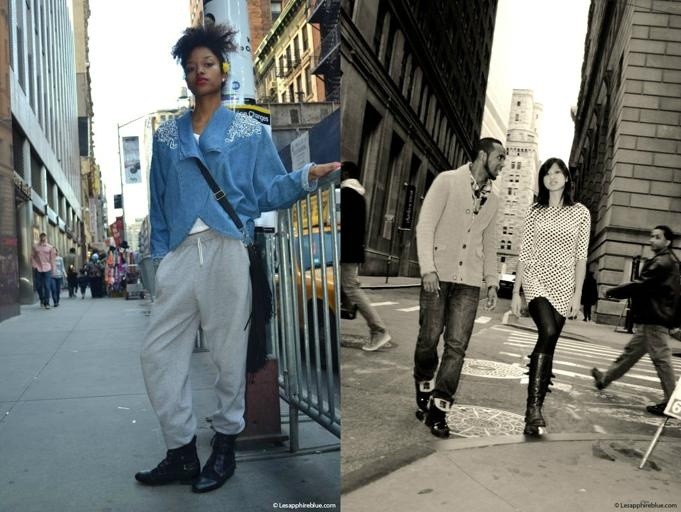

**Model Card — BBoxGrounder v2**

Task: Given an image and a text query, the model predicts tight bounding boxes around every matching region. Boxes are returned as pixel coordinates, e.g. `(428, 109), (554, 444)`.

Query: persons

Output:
(341, 161), (393, 352)
(509, 157), (591, 441)
(592, 223), (681, 420)
(29, 232), (56, 310)
(134, 17), (342, 493)
(203, 13), (216, 29)
(412, 135), (507, 442)
(581, 271), (598, 322)
(50, 243), (129, 308)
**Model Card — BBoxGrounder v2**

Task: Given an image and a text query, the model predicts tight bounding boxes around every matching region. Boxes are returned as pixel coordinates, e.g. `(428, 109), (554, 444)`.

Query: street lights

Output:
(117, 108), (180, 259)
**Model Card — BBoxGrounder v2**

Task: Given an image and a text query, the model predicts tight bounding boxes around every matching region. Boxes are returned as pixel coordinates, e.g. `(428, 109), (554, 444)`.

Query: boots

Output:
(192, 432), (239, 493)
(523, 352), (551, 427)
(135, 435), (200, 485)
(416, 389), (455, 438)
(523, 372), (555, 436)
(413, 374), (435, 415)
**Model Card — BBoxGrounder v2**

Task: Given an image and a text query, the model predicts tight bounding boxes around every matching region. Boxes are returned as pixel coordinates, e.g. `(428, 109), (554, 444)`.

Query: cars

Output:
(270, 226), (341, 372)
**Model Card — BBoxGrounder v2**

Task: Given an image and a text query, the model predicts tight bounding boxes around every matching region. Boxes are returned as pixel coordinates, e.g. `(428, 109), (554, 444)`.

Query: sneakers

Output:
(45, 304), (50, 309)
(647, 404), (674, 418)
(592, 368), (605, 390)
(361, 331), (392, 351)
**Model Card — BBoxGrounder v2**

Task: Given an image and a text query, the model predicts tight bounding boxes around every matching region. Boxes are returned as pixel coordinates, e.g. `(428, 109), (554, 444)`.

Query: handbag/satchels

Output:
(243, 243), (272, 375)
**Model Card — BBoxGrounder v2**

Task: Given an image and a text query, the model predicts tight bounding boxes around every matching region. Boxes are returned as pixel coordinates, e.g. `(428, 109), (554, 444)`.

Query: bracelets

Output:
(512, 290), (519, 294)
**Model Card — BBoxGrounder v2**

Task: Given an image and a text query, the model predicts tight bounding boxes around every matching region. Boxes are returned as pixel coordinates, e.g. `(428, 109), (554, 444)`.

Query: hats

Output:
(120, 241), (129, 248)
